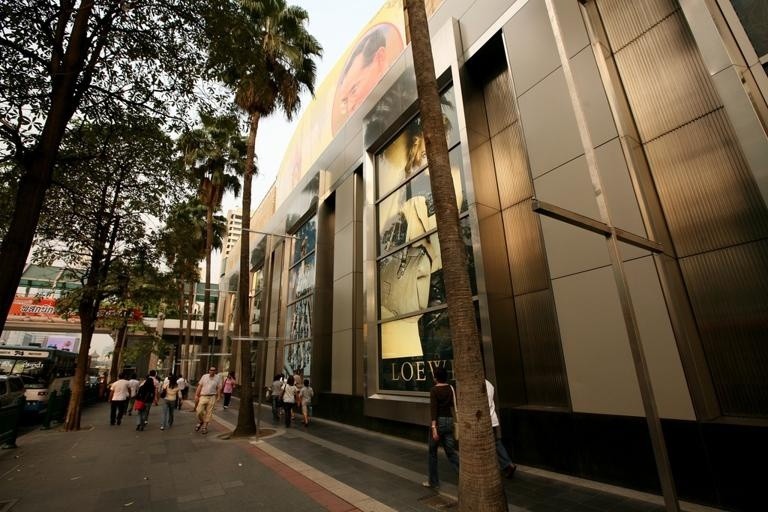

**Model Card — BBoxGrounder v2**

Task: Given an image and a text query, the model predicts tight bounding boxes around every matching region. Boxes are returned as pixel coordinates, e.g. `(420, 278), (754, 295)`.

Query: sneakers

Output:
(423, 480), (440, 488)
(136, 421), (148, 431)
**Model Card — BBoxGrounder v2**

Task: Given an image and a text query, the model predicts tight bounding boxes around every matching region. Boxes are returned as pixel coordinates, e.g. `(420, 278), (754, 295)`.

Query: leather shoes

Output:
(505, 464), (517, 478)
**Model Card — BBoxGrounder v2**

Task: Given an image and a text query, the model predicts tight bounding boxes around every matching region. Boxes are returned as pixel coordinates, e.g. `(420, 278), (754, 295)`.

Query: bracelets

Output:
(431, 426), (438, 429)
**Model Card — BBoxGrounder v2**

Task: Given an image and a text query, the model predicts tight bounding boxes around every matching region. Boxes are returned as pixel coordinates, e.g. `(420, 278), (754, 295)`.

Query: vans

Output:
(0, 374), (27, 418)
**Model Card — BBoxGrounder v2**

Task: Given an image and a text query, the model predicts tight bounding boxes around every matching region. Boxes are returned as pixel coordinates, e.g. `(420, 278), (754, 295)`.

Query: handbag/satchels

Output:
(134, 401), (144, 409)
(160, 389), (166, 398)
(275, 397), (284, 407)
(265, 393), (272, 403)
(453, 422), (460, 441)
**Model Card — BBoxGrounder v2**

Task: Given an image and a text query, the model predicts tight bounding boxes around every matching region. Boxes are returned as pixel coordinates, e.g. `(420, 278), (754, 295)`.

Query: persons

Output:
(420, 365), (460, 487)
(378, 115), (463, 361)
(338, 29), (392, 116)
(106, 364), (319, 437)
(484, 379), (517, 480)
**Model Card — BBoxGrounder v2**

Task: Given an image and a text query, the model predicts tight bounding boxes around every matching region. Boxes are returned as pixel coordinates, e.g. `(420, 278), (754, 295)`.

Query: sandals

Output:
(202, 428), (208, 434)
(195, 423), (201, 431)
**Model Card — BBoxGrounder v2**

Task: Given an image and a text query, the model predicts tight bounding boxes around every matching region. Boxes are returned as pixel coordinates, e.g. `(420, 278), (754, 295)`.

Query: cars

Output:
(88, 375), (98, 389)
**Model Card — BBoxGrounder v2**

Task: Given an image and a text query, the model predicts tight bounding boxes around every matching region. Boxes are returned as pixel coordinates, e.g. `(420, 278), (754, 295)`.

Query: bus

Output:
(0, 341), (92, 412)
